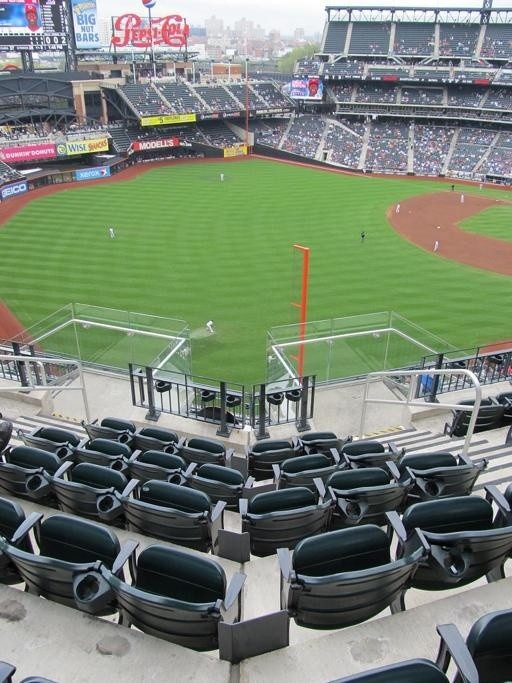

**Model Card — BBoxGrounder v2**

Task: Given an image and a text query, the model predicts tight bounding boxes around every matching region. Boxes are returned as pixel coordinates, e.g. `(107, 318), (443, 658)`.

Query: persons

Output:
(359, 231), (368, 241)
(109, 227), (114, 238)
(396, 204), (401, 214)
(205, 319), (215, 334)
(0, 29), (512, 202)
(433, 239), (439, 252)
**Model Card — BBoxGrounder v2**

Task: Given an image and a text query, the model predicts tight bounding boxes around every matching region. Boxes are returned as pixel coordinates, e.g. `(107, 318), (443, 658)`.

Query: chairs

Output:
(468, 609), (510, 683)
(0, 512), (121, 626)
(111, 531), (242, 653)
(0, 498), (32, 594)
(323, 622), (480, 682)
(384, 484), (511, 612)
(276, 524), (430, 631)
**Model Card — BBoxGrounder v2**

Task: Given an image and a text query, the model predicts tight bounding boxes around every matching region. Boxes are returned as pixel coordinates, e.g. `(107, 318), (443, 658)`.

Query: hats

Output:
(309, 79), (318, 86)
